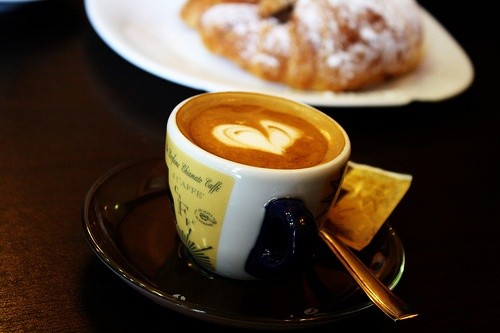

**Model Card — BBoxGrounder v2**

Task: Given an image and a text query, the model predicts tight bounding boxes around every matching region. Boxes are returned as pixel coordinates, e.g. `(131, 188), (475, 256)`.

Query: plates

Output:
(83, 156), (405, 330)
(84, 0), (475, 107)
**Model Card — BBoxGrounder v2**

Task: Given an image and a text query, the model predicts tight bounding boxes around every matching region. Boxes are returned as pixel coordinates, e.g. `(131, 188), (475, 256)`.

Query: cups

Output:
(165, 92), (352, 279)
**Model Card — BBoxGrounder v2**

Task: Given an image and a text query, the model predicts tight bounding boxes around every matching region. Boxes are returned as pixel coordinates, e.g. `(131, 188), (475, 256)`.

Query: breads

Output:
(179, 0), (425, 91)
